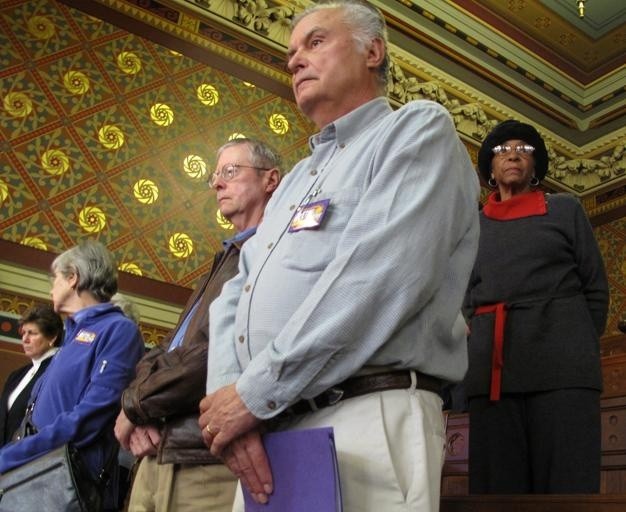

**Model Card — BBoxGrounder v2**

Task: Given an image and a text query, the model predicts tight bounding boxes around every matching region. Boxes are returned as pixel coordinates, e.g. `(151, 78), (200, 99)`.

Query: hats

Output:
(478, 119), (549, 190)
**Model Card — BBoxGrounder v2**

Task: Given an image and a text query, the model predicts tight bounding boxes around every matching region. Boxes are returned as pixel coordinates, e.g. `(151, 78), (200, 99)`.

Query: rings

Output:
(206, 424), (215, 437)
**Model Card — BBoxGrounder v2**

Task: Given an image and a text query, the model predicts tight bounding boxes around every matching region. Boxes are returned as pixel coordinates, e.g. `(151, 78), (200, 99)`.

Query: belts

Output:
(259, 368), (452, 434)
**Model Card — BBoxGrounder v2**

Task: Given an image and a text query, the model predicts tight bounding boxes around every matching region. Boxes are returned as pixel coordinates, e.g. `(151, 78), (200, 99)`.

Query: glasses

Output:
(208, 163), (270, 190)
(492, 143), (536, 157)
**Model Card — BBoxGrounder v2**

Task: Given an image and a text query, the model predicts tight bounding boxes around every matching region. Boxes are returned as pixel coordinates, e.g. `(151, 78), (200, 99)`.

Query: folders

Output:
(242, 427), (344, 511)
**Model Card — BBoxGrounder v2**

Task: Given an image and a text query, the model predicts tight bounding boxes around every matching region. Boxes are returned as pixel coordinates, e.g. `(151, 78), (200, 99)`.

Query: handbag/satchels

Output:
(0, 439), (106, 511)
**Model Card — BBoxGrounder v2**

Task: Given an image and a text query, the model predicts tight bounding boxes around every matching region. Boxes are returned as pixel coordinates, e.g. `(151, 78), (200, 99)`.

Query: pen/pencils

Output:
(100, 361), (107, 373)
(301, 194), (312, 214)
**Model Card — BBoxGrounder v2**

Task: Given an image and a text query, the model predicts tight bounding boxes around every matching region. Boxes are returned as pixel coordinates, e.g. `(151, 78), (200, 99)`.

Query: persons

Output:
(0, 238), (145, 512)
(199, 2), (483, 512)
(461, 118), (610, 495)
(113, 136), (283, 512)
(0, 303), (64, 450)
